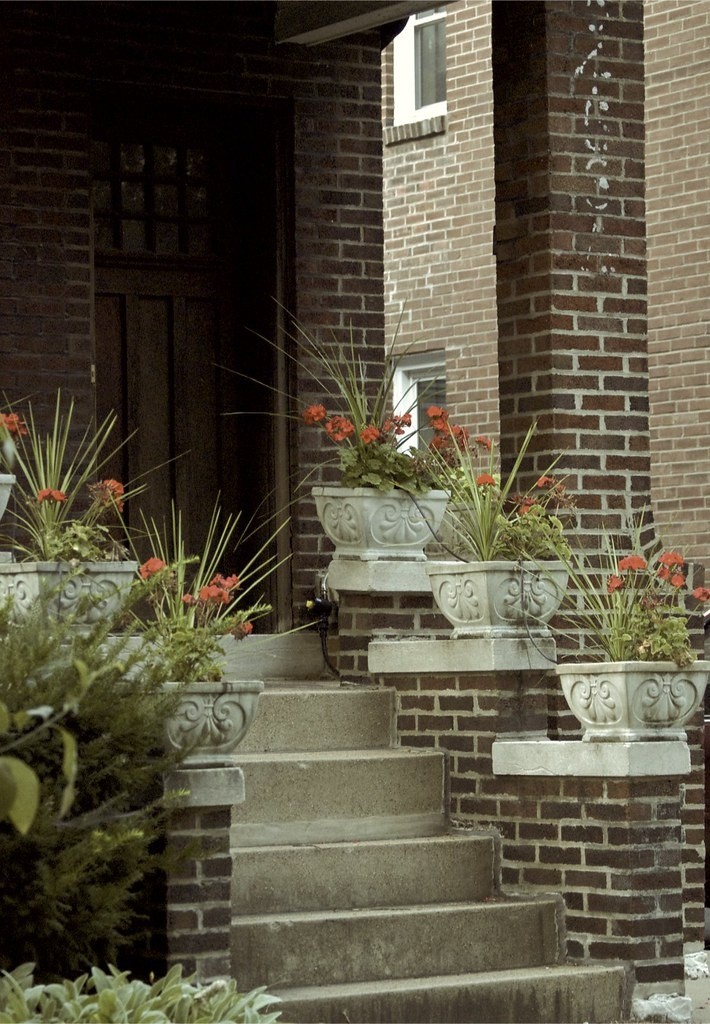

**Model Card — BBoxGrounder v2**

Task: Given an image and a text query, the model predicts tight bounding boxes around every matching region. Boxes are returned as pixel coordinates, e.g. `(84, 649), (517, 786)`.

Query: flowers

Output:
(510, 497), (710, 667)
(0, 412), (27, 475)
(71, 489), (333, 681)
(402, 405), (581, 564)
(211, 285), (454, 498)
(0, 387), (194, 563)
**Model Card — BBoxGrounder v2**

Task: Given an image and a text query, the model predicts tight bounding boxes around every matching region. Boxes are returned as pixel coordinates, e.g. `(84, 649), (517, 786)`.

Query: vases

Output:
(425, 558), (575, 638)
(0, 474), (17, 522)
(310, 486), (452, 562)
(109, 682), (267, 759)
(0, 561), (138, 634)
(555, 659), (710, 742)
